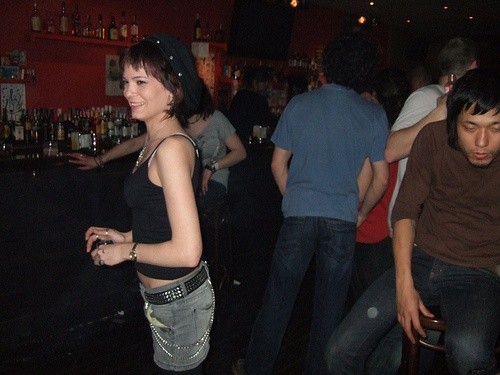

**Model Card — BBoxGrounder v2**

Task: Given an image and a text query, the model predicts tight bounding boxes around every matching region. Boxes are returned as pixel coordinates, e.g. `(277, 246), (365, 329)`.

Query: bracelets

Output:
(204, 164), (215, 174)
(211, 159), (219, 171)
(93, 155), (105, 167)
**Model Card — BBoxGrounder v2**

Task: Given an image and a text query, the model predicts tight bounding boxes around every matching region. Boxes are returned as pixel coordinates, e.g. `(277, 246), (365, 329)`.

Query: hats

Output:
(141, 32), (202, 114)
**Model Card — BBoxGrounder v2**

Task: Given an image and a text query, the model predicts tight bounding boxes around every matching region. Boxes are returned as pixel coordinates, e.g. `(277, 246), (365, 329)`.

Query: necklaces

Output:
(135, 144), (148, 167)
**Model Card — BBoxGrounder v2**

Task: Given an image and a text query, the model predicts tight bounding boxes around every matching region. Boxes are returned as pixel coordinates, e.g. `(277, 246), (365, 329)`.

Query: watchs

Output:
(130, 242), (140, 262)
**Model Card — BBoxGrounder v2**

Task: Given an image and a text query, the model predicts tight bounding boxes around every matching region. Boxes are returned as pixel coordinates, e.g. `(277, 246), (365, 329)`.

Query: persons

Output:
(84, 34), (217, 375)
(243, 39), (391, 375)
(230, 63), (277, 156)
(69, 75), (248, 294)
(325, 67), (500, 375)
(339, 36), (500, 375)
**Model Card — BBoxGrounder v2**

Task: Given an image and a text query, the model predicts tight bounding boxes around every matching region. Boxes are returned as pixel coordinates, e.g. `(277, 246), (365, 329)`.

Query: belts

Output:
(144, 265), (208, 305)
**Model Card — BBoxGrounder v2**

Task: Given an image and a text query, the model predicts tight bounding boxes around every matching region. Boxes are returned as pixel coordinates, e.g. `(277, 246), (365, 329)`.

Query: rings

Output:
(99, 260), (104, 265)
(102, 249), (105, 253)
(96, 234), (99, 239)
(79, 154), (83, 157)
(105, 229), (109, 235)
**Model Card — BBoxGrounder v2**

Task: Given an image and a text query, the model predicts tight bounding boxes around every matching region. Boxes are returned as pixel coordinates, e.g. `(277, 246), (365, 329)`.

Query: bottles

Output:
(108, 16), (118, 41)
(82, 14), (94, 37)
(95, 15), (106, 39)
(444, 73), (458, 94)
(222, 48), (326, 113)
(0, 103), (147, 157)
(71, 4), (81, 36)
(0, 48), (27, 80)
(30, 3), (42, 32)
(120, 12), (128, 42)
(194, 13), (202, 41)
(45, 9), (56, 34)
(215, 23), (226, 42)
(130, 15), (139, 42)
(59, 1), (69, 36)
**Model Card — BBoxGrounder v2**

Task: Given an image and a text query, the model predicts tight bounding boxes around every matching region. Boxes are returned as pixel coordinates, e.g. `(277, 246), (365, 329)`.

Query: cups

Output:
(95, 229), (115, 250)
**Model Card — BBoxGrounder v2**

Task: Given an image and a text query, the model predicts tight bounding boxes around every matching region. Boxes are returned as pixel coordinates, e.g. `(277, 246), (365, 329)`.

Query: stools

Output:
(202, 201), (231, 304)
(402, 316), (446, 375)
